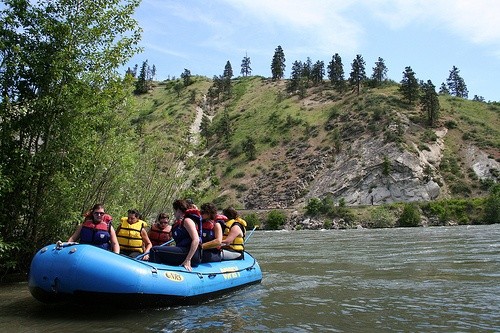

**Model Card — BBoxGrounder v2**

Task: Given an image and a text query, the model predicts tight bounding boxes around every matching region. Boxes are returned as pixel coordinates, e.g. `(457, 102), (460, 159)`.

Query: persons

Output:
(68, 204), (119, 254)
(116, 210), (152, 260)
(221, 206), (246, 260)
(200, 202), (223, 262)
(150, 200), (200, 271)
(148, 214), (172, 246)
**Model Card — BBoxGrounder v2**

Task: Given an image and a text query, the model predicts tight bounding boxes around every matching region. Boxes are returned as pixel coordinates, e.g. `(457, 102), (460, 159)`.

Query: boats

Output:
(28, 241), (262, 307)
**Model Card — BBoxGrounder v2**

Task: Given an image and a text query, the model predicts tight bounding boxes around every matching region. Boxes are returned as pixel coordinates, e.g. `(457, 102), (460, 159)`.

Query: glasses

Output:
(92, 212), (105, 216)
(201, 211), (206, 215)
(160, 221), (168, 225)
